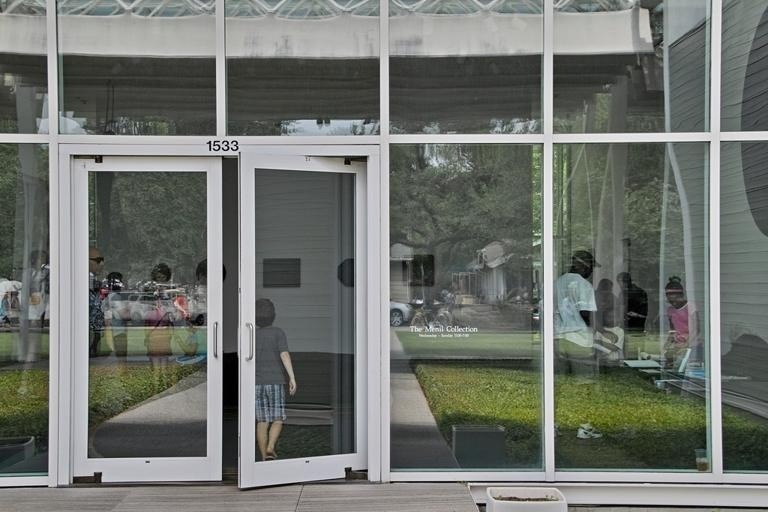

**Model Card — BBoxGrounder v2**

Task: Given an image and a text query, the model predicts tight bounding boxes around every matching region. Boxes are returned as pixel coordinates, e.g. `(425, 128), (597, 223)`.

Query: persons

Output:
(592, 273), (619, 327)
(594, 306), (624, 391)
(255, 297), (297, 460)
(13, 250), (208, 397)
(554, 249), (618, 438)
(618, 268), (648, 356)
(661, 275), (703, 368)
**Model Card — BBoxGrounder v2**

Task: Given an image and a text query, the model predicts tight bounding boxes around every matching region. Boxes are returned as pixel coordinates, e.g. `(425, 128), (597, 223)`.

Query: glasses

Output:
(89, 256), (105, 264)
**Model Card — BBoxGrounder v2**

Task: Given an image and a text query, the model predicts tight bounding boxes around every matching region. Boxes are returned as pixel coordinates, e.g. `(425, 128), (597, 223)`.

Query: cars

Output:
(100, 280), (207, 326)
(492, 286), (539, 323)
(390, 296), (414, 327)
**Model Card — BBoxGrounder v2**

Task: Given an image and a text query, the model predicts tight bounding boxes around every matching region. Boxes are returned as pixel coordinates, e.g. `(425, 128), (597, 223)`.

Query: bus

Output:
(13, 263), (95, 328)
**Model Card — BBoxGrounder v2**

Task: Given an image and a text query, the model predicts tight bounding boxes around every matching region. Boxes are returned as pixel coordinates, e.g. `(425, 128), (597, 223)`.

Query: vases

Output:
(452, 424), (507, 468)
(485, 487), (567, 512)
(1, 435), (36, 468)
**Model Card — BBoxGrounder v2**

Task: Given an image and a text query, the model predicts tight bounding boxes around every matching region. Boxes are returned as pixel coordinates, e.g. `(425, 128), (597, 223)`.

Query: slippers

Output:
(265, 455), (278, 461)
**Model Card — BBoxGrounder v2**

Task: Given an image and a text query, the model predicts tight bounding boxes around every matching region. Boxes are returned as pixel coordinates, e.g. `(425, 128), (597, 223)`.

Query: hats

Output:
(571, 250), (603, 268)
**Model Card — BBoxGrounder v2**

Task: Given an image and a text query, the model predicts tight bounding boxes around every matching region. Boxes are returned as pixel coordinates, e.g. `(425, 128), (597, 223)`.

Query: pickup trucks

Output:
(435, 291), (495, 332)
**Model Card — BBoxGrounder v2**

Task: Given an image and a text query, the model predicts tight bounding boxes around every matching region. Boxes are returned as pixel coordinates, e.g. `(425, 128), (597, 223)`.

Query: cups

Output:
(695, 448), (708, 472)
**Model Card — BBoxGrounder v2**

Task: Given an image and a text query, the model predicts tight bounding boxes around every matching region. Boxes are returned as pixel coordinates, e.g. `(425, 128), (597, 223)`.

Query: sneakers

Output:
(575, 426), (604, 440)
(554, 426), (563, 439)
